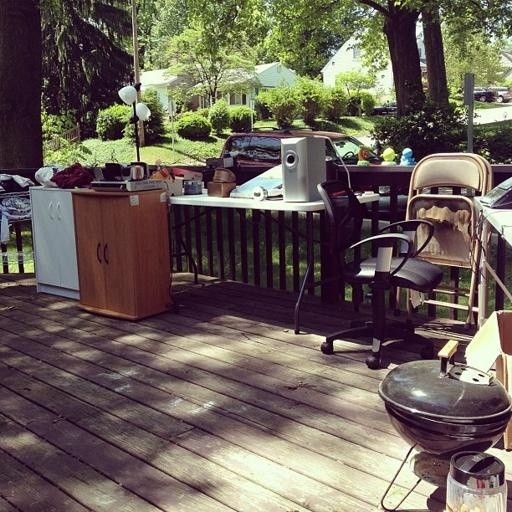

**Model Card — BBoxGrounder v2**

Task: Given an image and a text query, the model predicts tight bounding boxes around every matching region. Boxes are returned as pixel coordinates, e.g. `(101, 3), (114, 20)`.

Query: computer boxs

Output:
(280, 135), (328, 202)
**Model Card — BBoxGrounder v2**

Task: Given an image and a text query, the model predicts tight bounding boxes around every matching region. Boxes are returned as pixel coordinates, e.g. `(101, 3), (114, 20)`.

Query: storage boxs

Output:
(464, 311), (511, 452)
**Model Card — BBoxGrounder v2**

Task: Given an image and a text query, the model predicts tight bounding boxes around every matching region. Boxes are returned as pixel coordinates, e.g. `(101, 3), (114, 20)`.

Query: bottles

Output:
(152, 166), (171, 178)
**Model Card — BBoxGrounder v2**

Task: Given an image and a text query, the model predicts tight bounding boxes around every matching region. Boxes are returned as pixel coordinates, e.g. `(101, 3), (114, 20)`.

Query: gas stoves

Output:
(90, 179), (165, 191)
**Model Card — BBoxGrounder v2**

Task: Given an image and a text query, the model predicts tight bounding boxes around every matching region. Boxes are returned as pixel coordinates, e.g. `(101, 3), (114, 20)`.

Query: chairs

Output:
(394, 153), (494, 330)
(315, 178), (443, 370)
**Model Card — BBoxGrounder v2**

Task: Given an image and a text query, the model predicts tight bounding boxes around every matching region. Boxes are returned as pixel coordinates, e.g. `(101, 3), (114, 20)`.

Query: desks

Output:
(165, 189), (379, 336)
(331, 160), (512, 185)
(472, 195), (512, 330)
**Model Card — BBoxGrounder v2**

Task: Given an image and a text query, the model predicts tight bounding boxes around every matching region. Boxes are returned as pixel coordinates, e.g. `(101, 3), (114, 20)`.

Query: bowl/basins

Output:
(213, 168), (237, 184)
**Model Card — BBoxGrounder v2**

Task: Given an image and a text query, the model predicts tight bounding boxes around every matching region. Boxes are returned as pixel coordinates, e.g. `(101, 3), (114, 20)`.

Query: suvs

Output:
(218, 124), (400, 185)
(487, 86), (512, 104)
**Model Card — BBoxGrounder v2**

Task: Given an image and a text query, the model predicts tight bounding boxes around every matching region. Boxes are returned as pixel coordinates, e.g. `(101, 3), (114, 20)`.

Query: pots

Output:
(121, 164), (145, 181)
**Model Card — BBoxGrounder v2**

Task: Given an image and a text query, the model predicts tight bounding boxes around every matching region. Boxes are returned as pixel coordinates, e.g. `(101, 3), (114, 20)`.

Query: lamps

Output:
(118, 74), (151, 162)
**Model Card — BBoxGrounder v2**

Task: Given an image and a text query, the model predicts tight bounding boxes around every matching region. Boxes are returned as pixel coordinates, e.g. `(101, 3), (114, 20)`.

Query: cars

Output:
(372, 100), (397, 116)
(474, 87), (495, 103)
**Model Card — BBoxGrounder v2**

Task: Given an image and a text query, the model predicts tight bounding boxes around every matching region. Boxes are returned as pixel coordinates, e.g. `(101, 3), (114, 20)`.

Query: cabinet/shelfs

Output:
(28, 186), (81, 301)
(68, 189), (173, 321)
